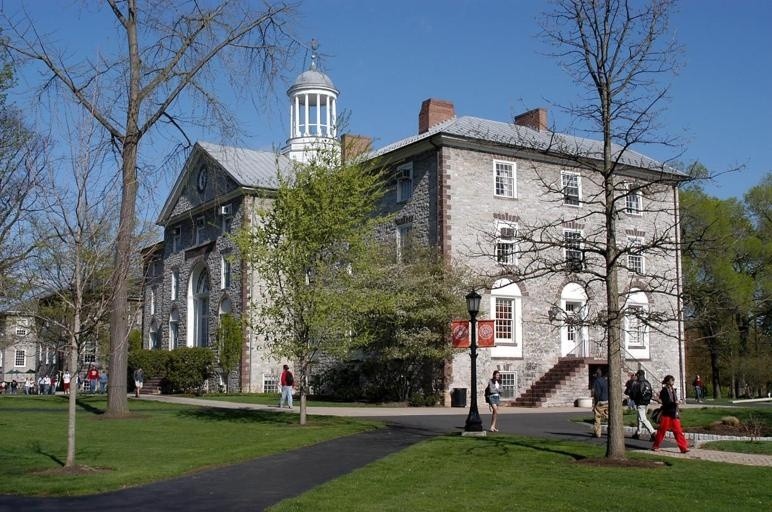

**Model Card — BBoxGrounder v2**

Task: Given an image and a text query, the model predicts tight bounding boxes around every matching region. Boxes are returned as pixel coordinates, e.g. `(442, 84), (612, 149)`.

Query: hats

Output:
(635, 370), (645, 376)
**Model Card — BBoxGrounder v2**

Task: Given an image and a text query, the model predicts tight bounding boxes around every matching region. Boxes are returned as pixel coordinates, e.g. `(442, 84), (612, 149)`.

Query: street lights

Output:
(465, 287), (482, 432)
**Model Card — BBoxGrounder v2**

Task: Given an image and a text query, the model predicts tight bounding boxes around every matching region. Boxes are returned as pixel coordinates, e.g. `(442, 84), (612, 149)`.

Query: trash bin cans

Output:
(40, 384), (49, 395)
(453, 388), (466, 407)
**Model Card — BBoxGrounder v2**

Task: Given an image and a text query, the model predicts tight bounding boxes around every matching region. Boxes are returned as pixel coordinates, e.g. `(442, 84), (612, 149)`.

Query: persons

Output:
(693, 374), (704, 403)
(134, 366), (144, 397)
(592, 367), (608, 437)
(280, 365), (294, 408)
(487, 370), (504, 432)
(0, 366), (108, 395)
(624, 370), (690, 453)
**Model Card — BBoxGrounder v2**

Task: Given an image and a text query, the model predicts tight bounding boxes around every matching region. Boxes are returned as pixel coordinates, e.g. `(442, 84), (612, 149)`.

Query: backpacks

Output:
(639, 381), (652, 401)
(485, 386), (490, 403)
(287, 372), (293, 386)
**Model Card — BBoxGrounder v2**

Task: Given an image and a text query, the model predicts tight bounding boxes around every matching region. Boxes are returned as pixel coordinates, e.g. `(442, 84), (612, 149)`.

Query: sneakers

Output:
(681, 450), (691, 453)
(490, 427), (499, 432)
(651, 447), (660, 451)
(632, 431), (642, 439)
(649, 432), (657, 442)
(597, 435), (601, 438)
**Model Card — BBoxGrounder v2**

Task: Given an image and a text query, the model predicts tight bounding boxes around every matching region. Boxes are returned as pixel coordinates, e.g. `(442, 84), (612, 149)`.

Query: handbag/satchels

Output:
(651, 406), (663, 424)
